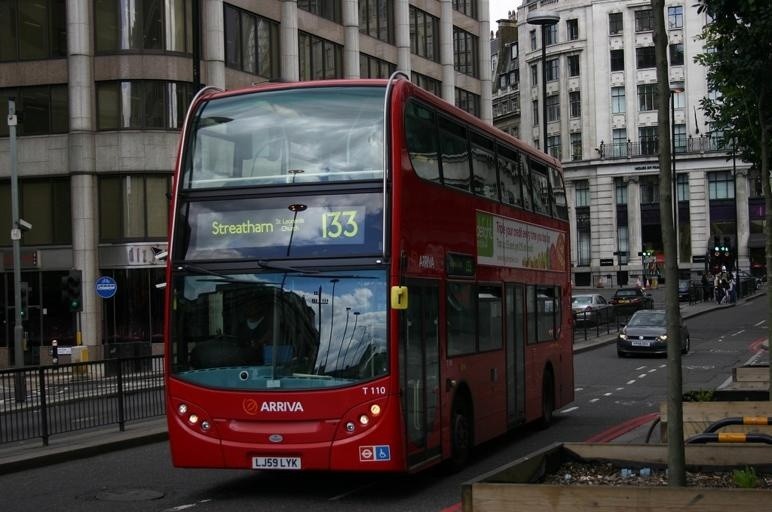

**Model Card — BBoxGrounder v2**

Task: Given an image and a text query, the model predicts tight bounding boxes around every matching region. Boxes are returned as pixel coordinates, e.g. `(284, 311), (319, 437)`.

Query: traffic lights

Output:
(713, 235), (721, 257)
(722, 235), (731, 257)
(638, 251), (651, 256)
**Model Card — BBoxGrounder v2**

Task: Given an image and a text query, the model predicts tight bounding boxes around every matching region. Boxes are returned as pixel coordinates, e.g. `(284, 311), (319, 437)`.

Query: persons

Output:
(701, 272), (737, 304)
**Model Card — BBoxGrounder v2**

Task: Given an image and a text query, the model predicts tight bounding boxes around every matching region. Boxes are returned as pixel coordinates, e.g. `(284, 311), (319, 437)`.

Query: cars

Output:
(570, 293), (616, 328)
(610, 287), (654, 315)
(678, 269), (761, 302)
(616, 309), (690, 357)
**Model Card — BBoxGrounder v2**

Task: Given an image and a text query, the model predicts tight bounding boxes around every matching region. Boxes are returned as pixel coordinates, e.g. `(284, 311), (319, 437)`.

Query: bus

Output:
(153, 71), (578, 482)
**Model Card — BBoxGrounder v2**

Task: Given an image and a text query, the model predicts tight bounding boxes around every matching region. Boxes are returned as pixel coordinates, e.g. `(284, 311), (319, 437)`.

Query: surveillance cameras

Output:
(18, 220), (32, 231)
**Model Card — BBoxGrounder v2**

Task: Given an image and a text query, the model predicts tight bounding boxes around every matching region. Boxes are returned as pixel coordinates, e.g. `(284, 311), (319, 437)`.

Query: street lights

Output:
(669, 86), (686, 261)
(525, 9), (561, 154)
